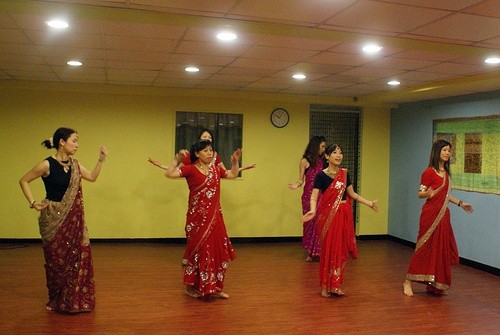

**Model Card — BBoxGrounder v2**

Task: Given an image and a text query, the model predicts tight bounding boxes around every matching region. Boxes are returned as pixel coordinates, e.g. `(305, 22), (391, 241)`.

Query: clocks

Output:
(270, 108), (290, 128)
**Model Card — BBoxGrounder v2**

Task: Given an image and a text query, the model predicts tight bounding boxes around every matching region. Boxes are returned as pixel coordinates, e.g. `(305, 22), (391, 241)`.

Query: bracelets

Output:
(458, 201), (463, 206)
(31, 201), (36, 208)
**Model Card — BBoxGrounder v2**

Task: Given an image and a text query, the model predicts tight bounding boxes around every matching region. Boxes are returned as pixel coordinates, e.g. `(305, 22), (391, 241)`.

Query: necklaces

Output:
(440, 169), (445, 172)
(52, 155), (70, 173)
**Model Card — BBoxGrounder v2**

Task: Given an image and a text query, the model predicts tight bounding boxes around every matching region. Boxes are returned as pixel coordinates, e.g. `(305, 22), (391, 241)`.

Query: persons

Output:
(301, 144), (378, 297)
(403, 140), (473, 296)
(147, 129), (257, 265)
(165, 140), (242, 297)
(19, 127), (107, 312)
(288, 135), (326, 262)
